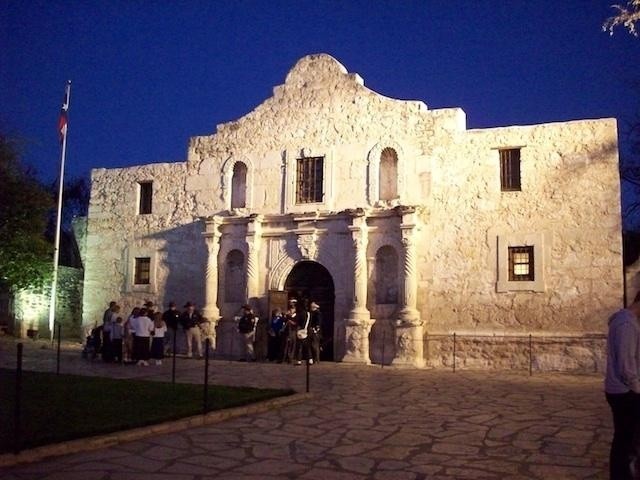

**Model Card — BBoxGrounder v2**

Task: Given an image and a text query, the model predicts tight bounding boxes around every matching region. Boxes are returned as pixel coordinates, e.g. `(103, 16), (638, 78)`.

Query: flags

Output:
(58, 86), (68, 145)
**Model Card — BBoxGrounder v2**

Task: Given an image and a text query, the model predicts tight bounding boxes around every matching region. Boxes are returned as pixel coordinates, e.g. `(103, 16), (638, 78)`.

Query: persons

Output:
(604, 290), (639, 479)
(84, 297), (322, 365)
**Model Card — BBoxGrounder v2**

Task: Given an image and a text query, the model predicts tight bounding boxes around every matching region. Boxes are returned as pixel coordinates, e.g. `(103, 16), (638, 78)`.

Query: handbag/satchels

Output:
(297, 329), (308, 339)
